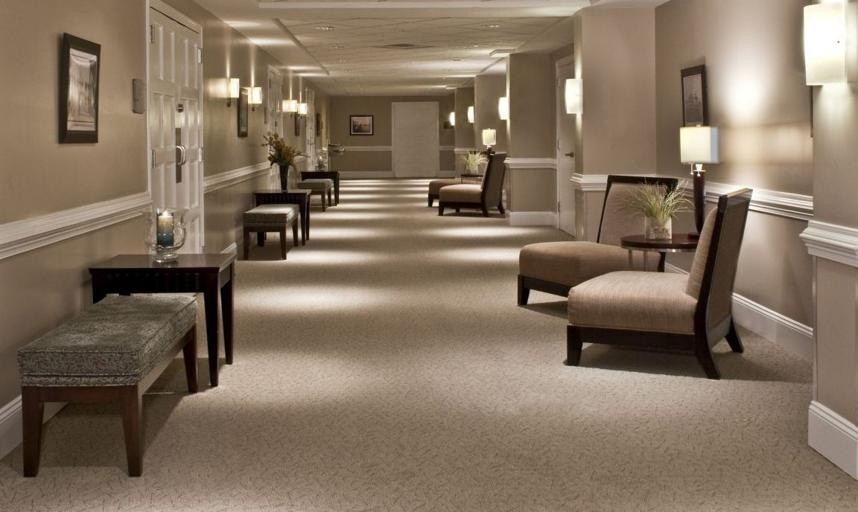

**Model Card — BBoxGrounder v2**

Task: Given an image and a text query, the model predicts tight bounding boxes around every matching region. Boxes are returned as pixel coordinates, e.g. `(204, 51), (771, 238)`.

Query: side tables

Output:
(460, 174), (483, 184)
(621, 233), (698, 273)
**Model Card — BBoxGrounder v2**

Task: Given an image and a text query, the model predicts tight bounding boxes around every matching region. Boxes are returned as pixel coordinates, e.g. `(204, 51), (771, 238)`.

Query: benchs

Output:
(297, 178), (333, 212)
(242, 203), (299, 260)
(16, 294), (199, 477)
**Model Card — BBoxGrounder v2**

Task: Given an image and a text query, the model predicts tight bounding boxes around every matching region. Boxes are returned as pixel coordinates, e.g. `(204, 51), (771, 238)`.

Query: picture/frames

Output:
(237, 86), (373, 137)
(57, 32), (101, 145)
(679, 63), (709, 126)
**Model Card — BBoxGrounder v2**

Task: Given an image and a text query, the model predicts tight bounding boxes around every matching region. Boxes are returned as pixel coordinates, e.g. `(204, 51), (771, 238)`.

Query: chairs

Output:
(560, 187), (754, 380)
(427, 150), (492, 207)
(438, 153), (509, 217)
(517, 174), (679, 306)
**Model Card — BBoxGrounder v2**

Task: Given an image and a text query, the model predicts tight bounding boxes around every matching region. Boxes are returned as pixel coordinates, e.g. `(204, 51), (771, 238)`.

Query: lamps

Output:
(802, 2), (858, 88)
(679, 125), (720, 241)
(482, 128), (497, 149)
(449, 77), (583, 126)
(230, 77), (308, 115)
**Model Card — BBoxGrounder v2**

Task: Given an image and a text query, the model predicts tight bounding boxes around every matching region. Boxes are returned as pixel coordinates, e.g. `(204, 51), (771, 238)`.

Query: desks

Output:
(88, 251), (238, 386)
(301, 170), (340, 206)
(251, 188), (312, 246)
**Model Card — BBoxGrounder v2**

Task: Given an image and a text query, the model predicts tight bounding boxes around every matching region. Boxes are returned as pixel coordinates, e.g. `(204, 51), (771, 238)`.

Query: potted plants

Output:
(460, 151), (488, 174)
(613, 175), (695, 240)
(261, 132), (311, 190)
